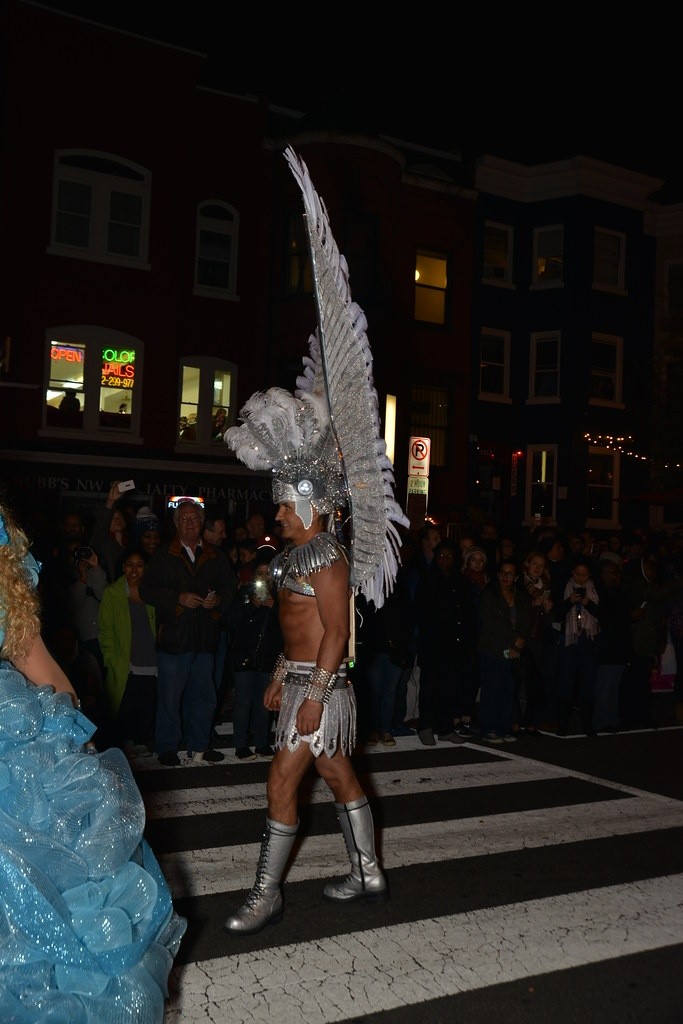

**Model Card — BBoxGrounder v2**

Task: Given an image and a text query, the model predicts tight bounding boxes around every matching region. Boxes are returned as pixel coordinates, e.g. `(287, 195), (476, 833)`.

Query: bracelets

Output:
(302, 666), (339, 703)
(270, 653), (288, 682)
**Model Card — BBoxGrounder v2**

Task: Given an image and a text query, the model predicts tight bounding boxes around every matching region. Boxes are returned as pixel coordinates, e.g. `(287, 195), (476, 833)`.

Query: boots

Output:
(224, 817), (301, 936)
(322, 794), (389, 904)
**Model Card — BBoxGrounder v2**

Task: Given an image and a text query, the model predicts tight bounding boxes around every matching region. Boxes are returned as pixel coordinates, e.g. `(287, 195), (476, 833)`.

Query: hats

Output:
(463, 545), (487, 568)
(223, 386), (348, 531)
(256, 536), (279, 551)
(133, 506), (156, 519)
(134, 518), (163, 535)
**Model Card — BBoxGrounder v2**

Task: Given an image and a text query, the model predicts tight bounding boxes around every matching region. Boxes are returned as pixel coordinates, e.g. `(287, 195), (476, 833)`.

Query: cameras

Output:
(117, 479), (136, 492)
(70, 544), (93, 570)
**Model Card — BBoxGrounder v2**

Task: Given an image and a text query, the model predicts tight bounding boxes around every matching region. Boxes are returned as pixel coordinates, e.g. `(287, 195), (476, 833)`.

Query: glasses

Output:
(499, 571), (516, 577)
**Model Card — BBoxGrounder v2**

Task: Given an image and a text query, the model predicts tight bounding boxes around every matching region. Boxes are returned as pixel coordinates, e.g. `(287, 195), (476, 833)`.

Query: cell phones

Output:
(577, 586), (587, 600)
(641, 601), (648, 609)
(542, 589), (551, 599)
(206, 589), (216, 598)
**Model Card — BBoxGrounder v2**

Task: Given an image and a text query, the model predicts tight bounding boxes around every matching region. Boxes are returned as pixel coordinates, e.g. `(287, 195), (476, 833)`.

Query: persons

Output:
(212, 409), (228, 439)
(59, 389), (80, 411)
(224, 455), (388, 934)
(347, 518), (682, 745)
(20, 480), (291, 765)
(0, 506), (188, 1024)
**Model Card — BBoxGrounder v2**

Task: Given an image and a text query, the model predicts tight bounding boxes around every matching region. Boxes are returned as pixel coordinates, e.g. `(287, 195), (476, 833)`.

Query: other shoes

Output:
(376, 731), (396, 746)
(437, 732), (465, 744)
(158, 752), (180, 766)
(129, 741), (152, 757)
(254, 746), (275, 760)
(450, 719), (540, 744)
(362, 732), (378, 745)
(234, 747), (257, 761)
(189, 748), (225, 762)
(419, 727), (436, 745)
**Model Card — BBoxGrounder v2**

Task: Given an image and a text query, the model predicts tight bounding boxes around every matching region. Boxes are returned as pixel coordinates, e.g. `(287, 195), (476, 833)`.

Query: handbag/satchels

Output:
(233, 647), (262, 671)
